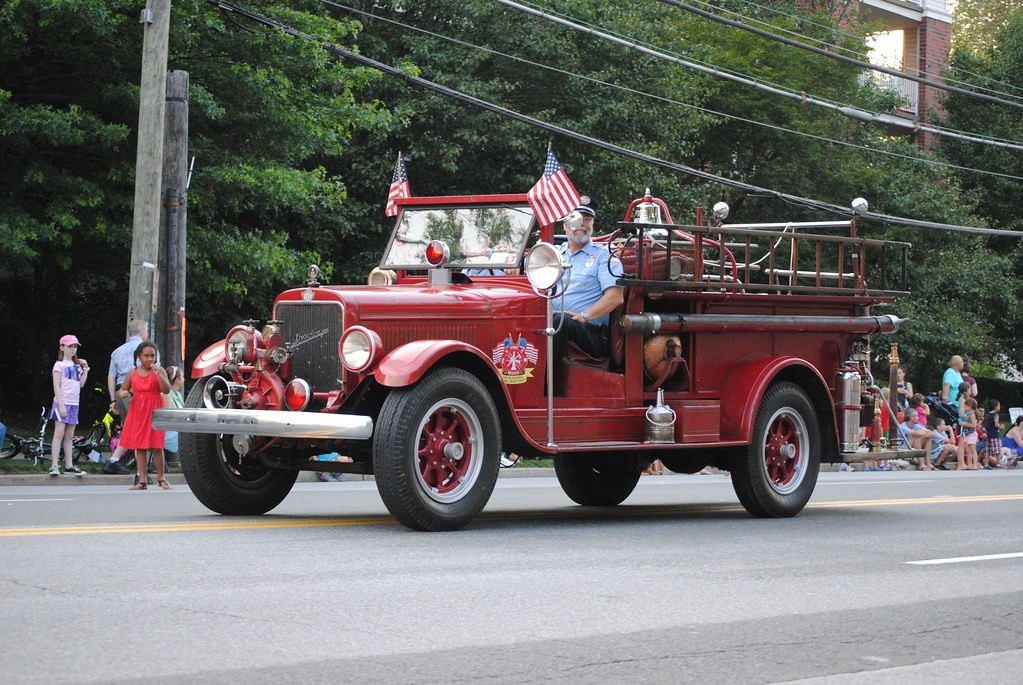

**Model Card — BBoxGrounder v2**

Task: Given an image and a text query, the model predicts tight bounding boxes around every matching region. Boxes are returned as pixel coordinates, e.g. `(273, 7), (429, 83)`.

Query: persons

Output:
(49, 335), (88, 476)
(118, 341), (172, 490)
(102, 319), (161, 475)
(503, 196), (623, 371)
(461, 233), (507, 276)
(500, 453), (522, 468)
(161, 366), (185, 468)
(642, 459), (712, 475)
(312, 452), (353, 482)
(839, 355), (1023, 472)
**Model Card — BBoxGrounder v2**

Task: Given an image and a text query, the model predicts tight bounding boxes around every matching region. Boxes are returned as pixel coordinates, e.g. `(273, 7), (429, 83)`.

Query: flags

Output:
(385, 155), (411, 218)
(527, 148), (582, 228)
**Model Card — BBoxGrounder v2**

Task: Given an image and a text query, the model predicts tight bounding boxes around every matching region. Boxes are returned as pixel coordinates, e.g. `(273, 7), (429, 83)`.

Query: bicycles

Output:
(0, 403), (94, 467)
(69, 387), (136, 467)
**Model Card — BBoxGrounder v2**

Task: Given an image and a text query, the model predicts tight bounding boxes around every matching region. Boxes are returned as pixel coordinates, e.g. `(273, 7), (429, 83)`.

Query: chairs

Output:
(559, 274), (644, 372)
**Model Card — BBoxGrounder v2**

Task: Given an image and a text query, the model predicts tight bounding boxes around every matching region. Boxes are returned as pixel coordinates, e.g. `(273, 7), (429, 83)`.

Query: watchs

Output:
(580, 312), (589, 320)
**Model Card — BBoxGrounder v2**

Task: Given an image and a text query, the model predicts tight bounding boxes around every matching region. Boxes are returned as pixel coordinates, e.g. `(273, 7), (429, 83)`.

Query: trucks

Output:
(151, 165), (915, 535)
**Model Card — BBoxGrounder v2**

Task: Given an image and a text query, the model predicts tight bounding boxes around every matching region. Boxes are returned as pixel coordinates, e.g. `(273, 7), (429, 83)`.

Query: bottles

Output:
(643, 388), (675, 443)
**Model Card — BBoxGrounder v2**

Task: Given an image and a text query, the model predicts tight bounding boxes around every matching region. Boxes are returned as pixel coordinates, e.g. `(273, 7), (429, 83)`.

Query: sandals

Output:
(129, 482), (147, 490)
(158, 479), (170, 490)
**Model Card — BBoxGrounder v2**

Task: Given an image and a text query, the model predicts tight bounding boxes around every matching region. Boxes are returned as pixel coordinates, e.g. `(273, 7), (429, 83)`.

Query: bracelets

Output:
(84, 367), (90, 371)
(111, 400), (116, 403)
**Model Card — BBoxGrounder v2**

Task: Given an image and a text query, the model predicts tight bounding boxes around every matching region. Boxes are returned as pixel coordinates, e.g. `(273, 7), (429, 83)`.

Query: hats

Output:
(59, 335), (81, 347)
(575, 195), (599, 218)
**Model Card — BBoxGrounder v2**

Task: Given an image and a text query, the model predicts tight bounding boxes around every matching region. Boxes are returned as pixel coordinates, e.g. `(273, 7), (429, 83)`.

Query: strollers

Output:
(925, 395), (987, 438)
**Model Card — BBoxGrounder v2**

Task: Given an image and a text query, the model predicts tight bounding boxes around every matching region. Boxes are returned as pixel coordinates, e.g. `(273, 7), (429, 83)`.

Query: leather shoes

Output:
(102, 460), (129, 474)
(134, 476), (154, 485)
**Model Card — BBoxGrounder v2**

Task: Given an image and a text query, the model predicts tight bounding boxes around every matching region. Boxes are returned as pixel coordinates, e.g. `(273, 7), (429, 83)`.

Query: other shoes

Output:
(333, 473), (345, 482)
(499, 455), (515, 467)
(695, 470), (713, 476)
(864, 462), (993, 471)
(639, 468), (664, 475)
(320, 472), (337, 482)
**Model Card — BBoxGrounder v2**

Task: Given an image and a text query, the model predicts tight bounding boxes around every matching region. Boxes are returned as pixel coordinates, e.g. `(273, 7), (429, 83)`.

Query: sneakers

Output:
(64, 465), (87, 476)
(49, 466), (59, 475)
(838, 465), (855, 473)
